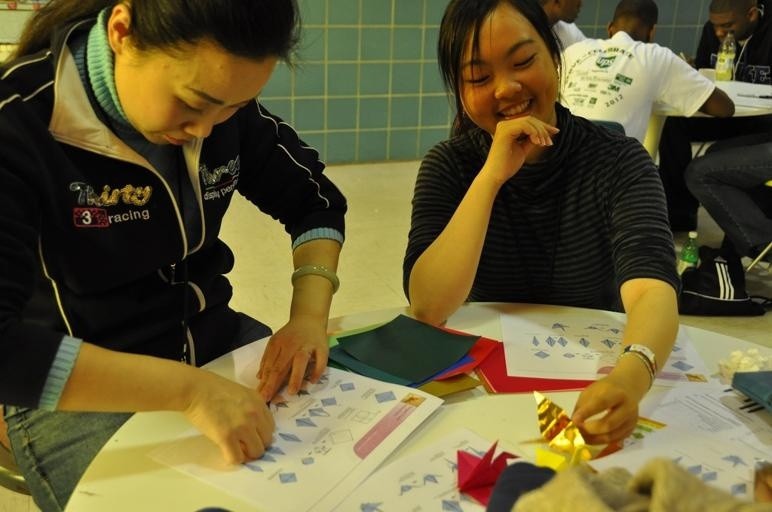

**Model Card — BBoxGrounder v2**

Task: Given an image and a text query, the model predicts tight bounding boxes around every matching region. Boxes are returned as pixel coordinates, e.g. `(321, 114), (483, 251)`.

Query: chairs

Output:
(742, 181), (772, 276)
(0, 411), (33, 511)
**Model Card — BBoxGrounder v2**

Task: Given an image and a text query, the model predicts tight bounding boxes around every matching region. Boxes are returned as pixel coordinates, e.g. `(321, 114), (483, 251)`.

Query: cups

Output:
(699, 69), (715, 81)
(715, 30), (737, 82)
(677, 231), (700, 278)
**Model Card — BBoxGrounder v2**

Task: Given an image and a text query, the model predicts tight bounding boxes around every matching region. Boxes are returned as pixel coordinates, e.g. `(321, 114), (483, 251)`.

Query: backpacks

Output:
(677, 245), (772, 316)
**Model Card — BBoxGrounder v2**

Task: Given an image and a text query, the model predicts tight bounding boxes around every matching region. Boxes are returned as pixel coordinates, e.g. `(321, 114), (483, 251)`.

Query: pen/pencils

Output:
(679, 52), (685, 61)
(739, 399), (763, 412)
(737, 93), (772, 98)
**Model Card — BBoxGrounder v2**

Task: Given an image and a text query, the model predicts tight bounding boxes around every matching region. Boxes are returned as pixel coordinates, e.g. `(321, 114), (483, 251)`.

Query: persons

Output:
(544, 0), (584, 49)
(556, 1), (736, 142)
(684, 141), (772, 270)
(402, 0), (678, 446)
(658, 1), (772, 232)
(0, 0), (347, 512)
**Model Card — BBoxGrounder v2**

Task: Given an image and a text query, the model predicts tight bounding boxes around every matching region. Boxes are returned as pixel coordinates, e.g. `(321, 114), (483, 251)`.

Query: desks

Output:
(60, 304), (771, 511)
(653, 81), (772, 142)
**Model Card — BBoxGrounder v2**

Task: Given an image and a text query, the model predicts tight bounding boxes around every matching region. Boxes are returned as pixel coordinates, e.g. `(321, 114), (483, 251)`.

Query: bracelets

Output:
(292, 264), (340, 294)
(616, 343), (658, 387)
